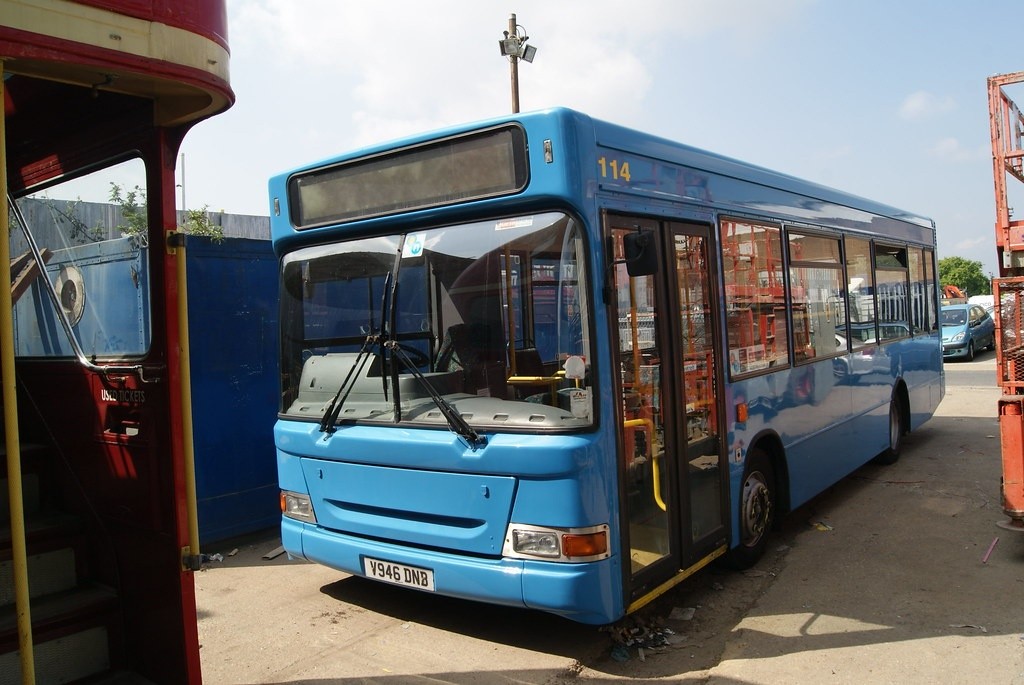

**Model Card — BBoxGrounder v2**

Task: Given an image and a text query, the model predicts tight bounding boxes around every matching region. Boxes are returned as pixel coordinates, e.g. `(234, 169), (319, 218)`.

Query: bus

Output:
(0, 0), (235, 684)
(267, 105), (945, 625)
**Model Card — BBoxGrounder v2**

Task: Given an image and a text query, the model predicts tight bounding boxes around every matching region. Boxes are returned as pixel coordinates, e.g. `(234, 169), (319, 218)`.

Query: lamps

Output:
(500, 39), (520, 56)
(522, 44), (537, 64)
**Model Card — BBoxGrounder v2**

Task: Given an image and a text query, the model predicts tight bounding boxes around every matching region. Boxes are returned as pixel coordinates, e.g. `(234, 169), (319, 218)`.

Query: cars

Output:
(940, 303), (996, 361)
